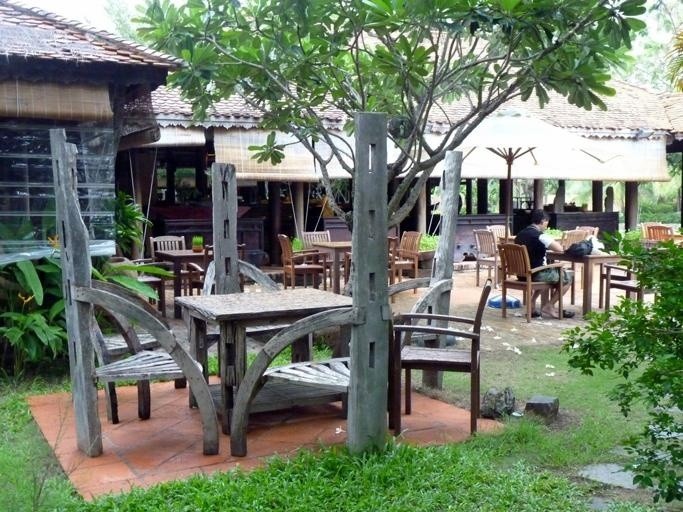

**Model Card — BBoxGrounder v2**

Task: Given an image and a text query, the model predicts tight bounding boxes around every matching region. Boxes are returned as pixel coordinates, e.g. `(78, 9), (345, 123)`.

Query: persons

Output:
(514, 209), (573, 320)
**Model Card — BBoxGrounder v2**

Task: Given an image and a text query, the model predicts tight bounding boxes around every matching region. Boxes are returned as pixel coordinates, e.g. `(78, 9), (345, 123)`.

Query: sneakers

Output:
(525, 310), (541, 318)
(563, 310), (575, 317)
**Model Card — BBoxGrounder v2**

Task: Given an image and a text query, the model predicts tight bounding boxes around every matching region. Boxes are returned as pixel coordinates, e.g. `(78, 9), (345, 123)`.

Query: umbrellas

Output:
(384, 113), (627, 227)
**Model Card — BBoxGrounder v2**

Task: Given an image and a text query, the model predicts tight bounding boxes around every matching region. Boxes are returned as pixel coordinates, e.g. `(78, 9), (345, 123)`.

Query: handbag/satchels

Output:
(566, 237), (592, 257)
(546, 268), (568, 282)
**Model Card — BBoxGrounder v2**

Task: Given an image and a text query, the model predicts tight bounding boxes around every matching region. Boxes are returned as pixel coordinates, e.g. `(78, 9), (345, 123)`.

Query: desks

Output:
(173, 287), (354, 435)
(155, 249), (214, 319)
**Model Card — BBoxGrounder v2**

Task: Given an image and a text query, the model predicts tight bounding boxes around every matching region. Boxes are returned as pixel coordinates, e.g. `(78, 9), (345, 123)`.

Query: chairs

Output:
(473, 222), (673, 322)
(148, 235), (187, 310)
(186, 243), (244, 297)
(49, 127), (221, 458)
(389, 278), (492, 435)
(277, 230), (421, 292)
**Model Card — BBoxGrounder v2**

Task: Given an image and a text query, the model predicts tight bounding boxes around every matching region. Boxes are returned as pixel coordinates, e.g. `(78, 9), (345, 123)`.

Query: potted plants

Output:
(418, 235), (437, 269)
(192, 236), (203, 251)
